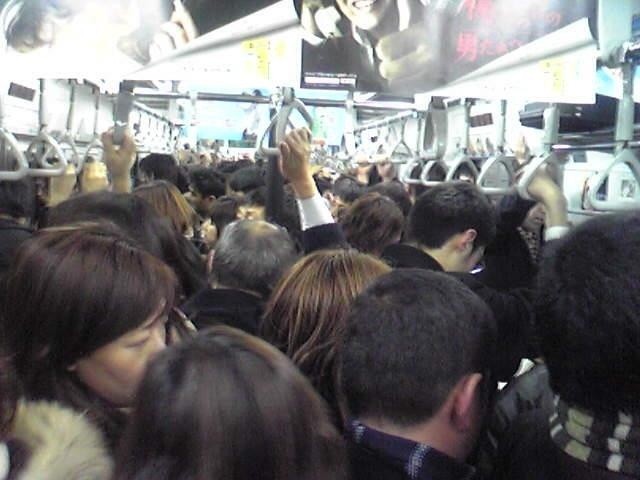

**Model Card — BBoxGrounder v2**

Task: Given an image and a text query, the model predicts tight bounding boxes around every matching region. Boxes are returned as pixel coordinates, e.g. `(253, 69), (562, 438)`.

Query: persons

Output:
(1, 127), (639, 479)
(301, 0), (447, 94)
